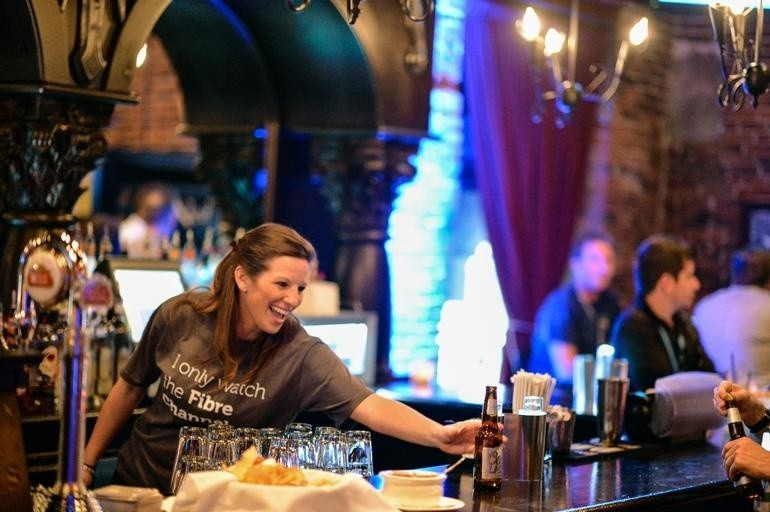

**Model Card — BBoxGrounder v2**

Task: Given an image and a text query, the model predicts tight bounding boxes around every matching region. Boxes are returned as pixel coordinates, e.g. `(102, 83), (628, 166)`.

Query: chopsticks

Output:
(510, 370), (556, 411)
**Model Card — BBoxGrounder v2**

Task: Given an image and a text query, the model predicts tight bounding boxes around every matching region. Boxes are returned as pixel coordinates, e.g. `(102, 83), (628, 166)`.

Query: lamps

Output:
(703, 1), (770, 114)
(512, 0), (651, 131)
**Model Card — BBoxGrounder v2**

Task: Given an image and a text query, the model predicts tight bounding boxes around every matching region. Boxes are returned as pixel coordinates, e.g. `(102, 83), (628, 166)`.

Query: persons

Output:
(609, 231), (717, 392)
(83, 223), (508, 493)
(687, 245), (770, 391)
(706, 379), (770, 511)
(529, 229), (620, 411)
(118, 185), (169, 256)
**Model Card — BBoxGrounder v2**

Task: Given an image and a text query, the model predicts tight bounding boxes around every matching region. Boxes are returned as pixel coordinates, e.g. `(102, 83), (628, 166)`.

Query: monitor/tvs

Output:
(93, 258), (188, 346)
(295, 311), (378, 385)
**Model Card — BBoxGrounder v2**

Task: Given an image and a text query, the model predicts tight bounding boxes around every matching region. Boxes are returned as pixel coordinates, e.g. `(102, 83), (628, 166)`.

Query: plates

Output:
(376, 495), (465, 511)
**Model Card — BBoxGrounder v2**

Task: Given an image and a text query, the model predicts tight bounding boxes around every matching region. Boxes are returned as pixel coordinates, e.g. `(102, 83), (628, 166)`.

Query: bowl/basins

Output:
(380, 468), (447, 507)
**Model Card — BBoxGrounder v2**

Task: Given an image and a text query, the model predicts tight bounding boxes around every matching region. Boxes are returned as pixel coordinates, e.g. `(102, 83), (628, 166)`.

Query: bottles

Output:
(474, 386), (503, 492)
(724, 399), (764, 501)
(70, 222), (131, 413)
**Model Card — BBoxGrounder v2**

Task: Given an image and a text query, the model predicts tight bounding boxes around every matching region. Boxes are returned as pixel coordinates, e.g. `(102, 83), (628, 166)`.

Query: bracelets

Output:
(744, 410), (770, 435)
(85, 462), (100, 476)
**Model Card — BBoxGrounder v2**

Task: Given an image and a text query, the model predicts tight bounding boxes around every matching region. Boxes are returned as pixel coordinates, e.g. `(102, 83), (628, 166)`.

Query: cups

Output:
(505, 411), (544, 483)
(546, 405), (576, 455)
(169, 423), (375, 496)
(598, 378), (629, 450)
